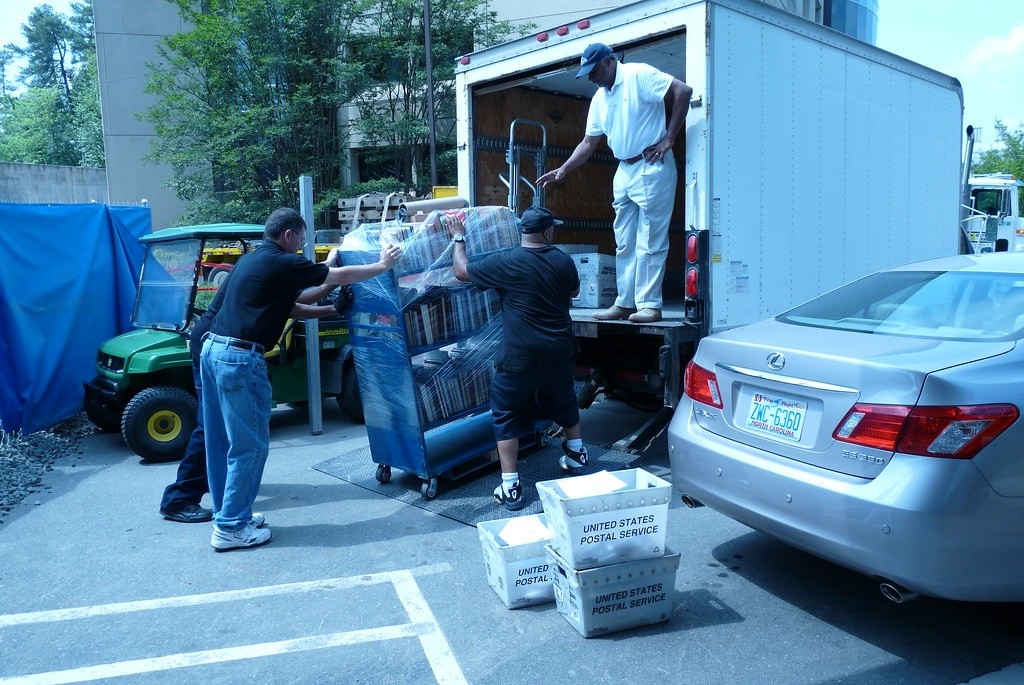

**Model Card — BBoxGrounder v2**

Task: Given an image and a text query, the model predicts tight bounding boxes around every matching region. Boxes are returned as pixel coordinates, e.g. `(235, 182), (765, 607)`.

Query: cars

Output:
(667, 251), (1024, 605)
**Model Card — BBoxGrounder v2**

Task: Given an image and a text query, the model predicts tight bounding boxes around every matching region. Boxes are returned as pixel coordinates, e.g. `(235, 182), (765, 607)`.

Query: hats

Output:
(519, 207), (564, 234)
(575, 43), (613, 79)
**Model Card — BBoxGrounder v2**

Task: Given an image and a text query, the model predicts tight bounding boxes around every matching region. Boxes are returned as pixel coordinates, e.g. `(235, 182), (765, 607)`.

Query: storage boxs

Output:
(534, 466), (671, 569)
(549, 243), (599, 260)
(571, 251), (618, 308)
(476, 513), (557, 609)
(544, 544), (682, 638)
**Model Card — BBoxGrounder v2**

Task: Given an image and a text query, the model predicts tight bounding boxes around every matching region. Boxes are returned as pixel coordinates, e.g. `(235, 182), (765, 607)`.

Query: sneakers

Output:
(160, 502), (212, 522)
(592, 303), (637, 320)
(493, 479), (524, 511)
(213, 512), (266, 529)
(559, 445), (588, 474)
(211, 521), (271, 549)
(628, 306), (662, 322)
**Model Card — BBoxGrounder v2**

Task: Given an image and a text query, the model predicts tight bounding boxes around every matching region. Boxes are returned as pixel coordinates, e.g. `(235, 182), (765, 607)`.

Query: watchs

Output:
(453, 233), (466, 243)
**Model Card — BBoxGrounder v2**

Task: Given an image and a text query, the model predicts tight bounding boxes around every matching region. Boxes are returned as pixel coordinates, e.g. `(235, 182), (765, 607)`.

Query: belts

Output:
(207, 334), (265, 356)
(623, 143), (661, 165)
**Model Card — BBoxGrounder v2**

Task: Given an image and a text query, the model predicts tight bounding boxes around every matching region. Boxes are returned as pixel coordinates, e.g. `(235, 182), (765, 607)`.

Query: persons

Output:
(445, 206), (590, 512)
(535, 42), (694, 325)
(159, 208), (402, 547)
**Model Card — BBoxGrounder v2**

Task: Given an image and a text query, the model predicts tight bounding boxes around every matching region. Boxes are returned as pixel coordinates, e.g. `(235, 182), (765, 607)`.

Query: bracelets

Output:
(333, 304), (339, 315)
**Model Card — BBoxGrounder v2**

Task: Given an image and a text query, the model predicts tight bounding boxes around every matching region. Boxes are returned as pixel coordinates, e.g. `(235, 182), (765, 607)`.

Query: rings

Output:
(656, 153), (659, 156)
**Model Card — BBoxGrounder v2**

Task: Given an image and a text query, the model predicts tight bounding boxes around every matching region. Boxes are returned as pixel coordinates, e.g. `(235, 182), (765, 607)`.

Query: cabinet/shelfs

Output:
(335, 224), (549, 499)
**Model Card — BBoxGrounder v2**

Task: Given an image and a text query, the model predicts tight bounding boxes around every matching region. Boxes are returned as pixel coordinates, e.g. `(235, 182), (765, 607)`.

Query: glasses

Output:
(290, 229), (308, 247)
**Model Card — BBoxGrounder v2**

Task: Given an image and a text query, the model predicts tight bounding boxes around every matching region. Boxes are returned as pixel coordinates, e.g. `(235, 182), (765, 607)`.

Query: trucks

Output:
(455, 1), (1024, 414)
